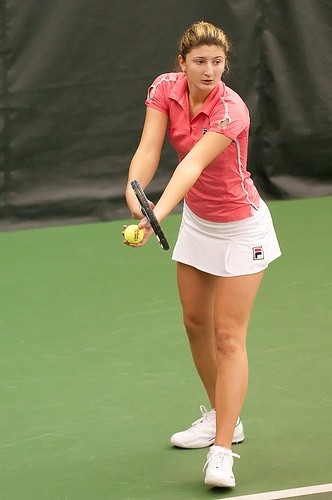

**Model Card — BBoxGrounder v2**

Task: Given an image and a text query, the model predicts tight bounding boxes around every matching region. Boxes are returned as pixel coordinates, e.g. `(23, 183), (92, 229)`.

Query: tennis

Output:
(124, 225), (144, 245)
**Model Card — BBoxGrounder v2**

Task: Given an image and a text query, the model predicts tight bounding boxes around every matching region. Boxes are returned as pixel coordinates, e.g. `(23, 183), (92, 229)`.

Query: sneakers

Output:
(203, 446), (241, 487)
(170, 406), (245, 449)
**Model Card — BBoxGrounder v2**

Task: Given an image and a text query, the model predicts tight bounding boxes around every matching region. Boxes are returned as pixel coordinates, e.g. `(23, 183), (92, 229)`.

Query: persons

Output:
(124, 20), (280, 490)
